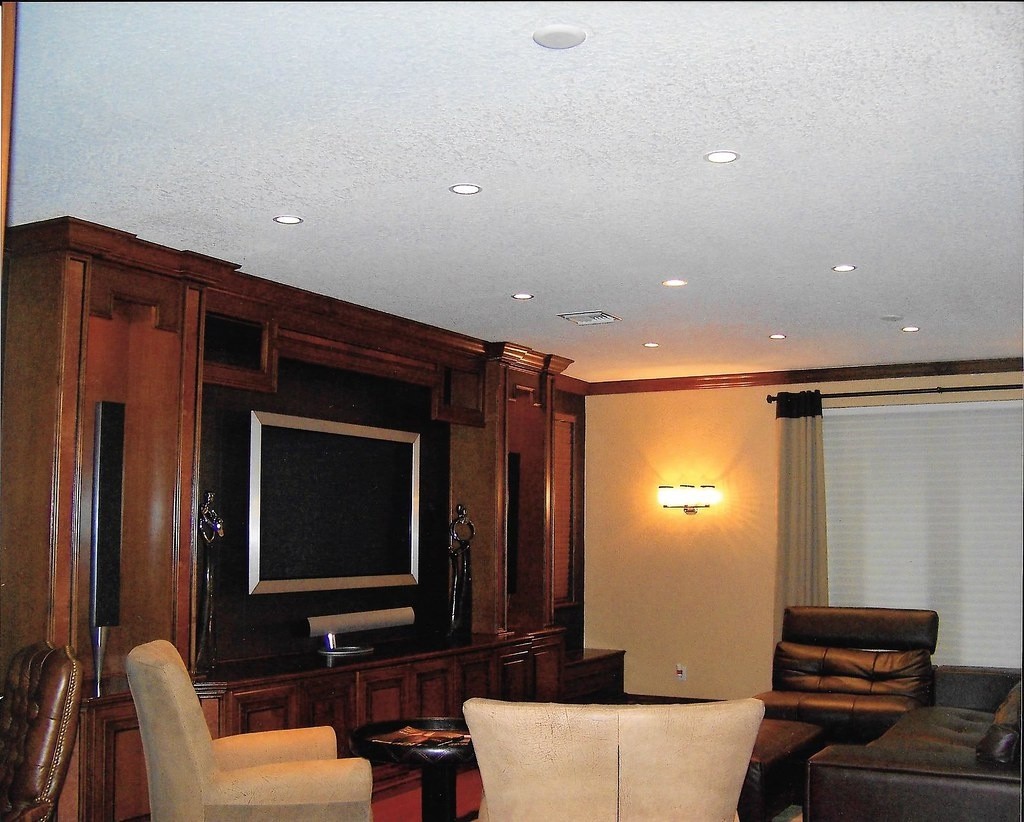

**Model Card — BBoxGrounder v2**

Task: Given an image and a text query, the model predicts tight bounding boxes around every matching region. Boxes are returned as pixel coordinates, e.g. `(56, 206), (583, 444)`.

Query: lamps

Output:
(658, 485), (716, 515)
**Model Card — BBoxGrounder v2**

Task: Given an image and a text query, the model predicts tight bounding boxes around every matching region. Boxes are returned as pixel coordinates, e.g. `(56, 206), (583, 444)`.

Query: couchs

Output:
(802, 662), (1024, 822)
(752, 606), (939, 745)
(126, 639), (377, 822)
(463, 696), (766, 822)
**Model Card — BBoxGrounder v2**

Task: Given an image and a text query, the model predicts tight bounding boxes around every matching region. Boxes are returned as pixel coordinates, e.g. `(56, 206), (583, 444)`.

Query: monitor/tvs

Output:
(246, 410), (422, 595)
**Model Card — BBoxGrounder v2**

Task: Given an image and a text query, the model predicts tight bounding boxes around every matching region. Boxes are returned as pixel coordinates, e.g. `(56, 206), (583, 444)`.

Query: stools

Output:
(735, 718), (826, 822)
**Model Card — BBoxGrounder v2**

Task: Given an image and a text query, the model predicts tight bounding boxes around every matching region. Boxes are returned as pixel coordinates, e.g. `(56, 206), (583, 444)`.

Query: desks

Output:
(348, 717), (479, 822)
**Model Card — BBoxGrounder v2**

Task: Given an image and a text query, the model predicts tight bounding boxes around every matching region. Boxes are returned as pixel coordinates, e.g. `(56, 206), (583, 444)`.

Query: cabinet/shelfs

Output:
(0, 216), (627, 822)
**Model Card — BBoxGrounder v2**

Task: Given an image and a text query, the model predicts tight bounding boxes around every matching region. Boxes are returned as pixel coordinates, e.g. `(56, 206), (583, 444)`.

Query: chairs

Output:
(0, 644), (83, 822)
